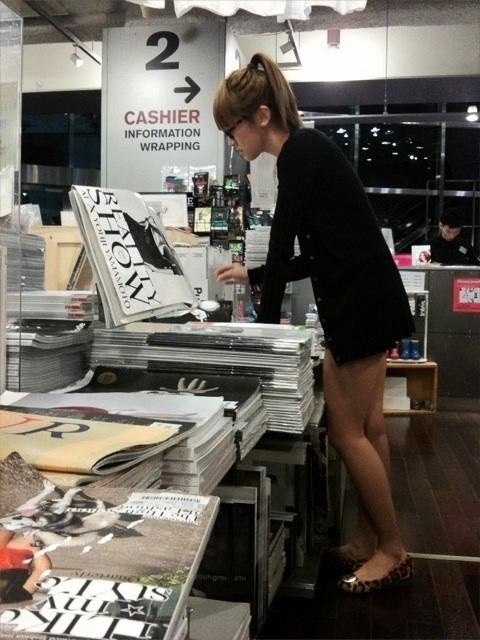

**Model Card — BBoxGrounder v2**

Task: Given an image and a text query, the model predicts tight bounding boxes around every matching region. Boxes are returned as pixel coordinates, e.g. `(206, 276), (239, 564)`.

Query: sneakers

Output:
(312, 541), (364, 569)
(333, 554), (414, 596)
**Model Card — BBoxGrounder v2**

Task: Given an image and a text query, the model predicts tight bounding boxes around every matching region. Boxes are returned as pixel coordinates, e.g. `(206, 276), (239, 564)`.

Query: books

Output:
(0, 162), (411, 639)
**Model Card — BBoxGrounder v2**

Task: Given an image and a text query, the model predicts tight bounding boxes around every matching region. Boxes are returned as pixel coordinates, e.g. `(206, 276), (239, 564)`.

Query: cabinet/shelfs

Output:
(382, 290), (438, 416)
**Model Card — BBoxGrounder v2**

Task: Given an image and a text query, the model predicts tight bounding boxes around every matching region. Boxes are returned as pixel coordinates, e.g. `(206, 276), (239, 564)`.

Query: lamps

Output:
(280, 28), (296, 56)
(69, 42), (85, 69)
(325, 29), (342, 51)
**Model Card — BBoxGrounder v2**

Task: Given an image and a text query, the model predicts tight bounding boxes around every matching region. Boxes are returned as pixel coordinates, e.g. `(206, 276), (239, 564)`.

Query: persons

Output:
(419, 251), (432, 264)
(410, 205), (478, 266)
(211, 51), (418, 597)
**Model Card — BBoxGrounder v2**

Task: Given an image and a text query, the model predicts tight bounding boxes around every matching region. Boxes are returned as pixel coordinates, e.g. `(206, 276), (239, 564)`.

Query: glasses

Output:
(223, 106), (258, 142)
